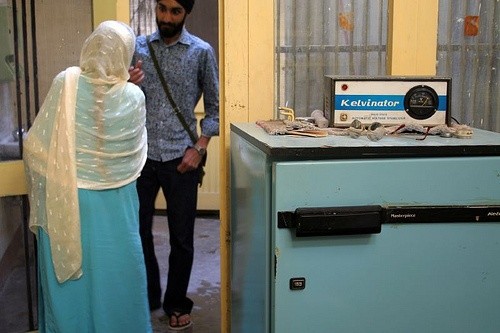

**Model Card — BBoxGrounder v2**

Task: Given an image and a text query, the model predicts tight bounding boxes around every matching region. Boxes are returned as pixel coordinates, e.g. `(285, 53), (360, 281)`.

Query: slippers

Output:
(168, 311), (192, 330)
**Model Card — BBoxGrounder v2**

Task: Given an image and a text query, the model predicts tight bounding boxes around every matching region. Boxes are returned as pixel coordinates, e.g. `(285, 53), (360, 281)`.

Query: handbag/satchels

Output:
(146, 35), (207, 187)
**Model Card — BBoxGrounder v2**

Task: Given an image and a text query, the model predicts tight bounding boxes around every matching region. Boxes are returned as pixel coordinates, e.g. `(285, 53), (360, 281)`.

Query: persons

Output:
(129, 0), (219, 331)
(23, 20), (152, 333)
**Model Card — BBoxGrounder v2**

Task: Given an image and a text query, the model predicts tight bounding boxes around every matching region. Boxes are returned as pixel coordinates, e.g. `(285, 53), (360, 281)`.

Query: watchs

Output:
(193, 145), (207, 155)
